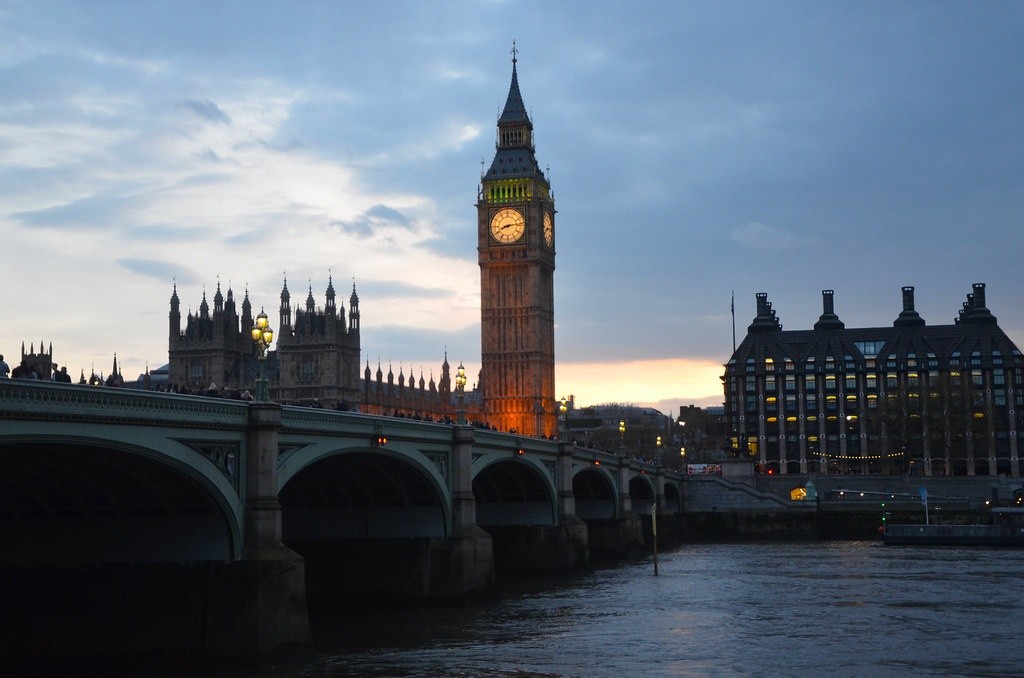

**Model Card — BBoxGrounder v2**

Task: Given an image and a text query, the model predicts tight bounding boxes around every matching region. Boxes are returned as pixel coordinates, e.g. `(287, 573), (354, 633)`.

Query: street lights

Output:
(655, 433), (663, 464)
(679, 447), (686, 472)
(250, 306), (274, 402)
(618, 420), (627, 456)
(455, 360), (470, 426)
(558, 397), (571, 441)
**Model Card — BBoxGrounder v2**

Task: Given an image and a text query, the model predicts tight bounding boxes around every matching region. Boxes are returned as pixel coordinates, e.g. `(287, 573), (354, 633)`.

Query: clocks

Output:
(542, 210), (554, 248)
(489, 207), (526, 245)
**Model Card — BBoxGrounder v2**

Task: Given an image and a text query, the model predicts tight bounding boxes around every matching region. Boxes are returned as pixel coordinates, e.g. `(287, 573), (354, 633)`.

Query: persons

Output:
(0, 354), (253, 401)
(282, 399), (681, 473)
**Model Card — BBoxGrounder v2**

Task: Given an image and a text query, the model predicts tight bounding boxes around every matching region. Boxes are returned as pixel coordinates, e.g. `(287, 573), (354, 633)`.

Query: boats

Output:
(881, 489), (1024, 546)
